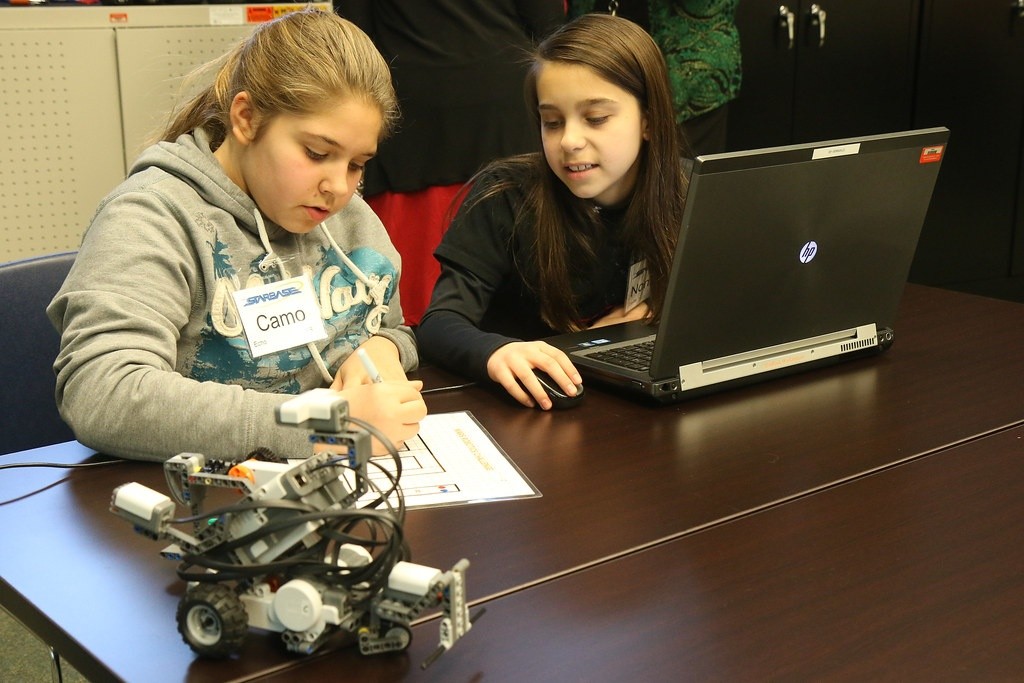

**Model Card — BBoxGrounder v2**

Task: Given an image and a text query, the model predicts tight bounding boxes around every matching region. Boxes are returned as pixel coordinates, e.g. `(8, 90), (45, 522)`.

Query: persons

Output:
(417, 15), (696, 410)
(46, 10), (427, 458)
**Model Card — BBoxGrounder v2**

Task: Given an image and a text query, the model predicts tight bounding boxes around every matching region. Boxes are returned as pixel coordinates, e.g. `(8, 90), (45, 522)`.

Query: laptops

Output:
(543, 126), (952, 397)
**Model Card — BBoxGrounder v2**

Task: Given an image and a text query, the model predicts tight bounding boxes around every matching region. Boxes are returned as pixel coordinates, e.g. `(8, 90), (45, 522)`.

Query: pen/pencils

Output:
(358, 346), (383, 385)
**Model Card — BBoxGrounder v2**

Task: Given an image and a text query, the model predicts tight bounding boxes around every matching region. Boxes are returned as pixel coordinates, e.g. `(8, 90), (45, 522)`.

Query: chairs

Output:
(0, 249), (79, 683)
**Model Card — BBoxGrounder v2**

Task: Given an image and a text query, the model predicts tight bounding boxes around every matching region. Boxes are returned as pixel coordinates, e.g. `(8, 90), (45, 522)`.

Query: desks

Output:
(1, 283), (1024, 683)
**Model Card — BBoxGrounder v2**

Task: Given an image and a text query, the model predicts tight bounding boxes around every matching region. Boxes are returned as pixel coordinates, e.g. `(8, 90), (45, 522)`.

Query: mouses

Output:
(516, 367), (584, 408)
